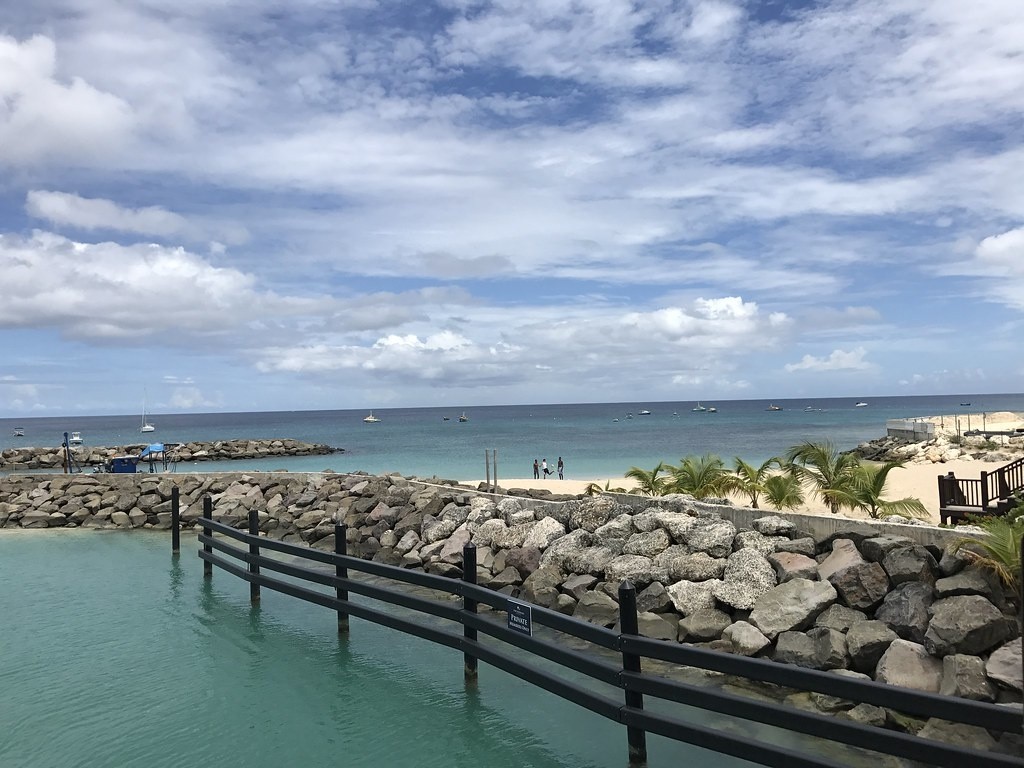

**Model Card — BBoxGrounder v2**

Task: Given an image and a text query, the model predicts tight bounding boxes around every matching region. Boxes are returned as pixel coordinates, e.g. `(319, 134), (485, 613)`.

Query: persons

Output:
(533, 459), (540, 479)
(539, 459), (555, 480)
(557, 456), (564, 480)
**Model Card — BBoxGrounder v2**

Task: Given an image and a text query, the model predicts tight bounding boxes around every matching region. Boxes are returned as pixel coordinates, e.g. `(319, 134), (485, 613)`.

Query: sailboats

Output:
(138, 385), (157, 433)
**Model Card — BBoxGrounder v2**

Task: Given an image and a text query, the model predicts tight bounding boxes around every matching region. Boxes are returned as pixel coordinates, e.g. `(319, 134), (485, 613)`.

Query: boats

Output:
(13, 426), (25, 437)
(363, 410), (382, 423)
(692, 401), (706, 412)
(459, 410), (468, 423)
(960, 399), (971, 407)
(708, 407), (717, 413)
(764, 403), (783, 411)
(69, 431), (83, 444)
(59, 432), (178, 473)
(855, 399), (868, 407)
(637, 409), (650, 415)
(804, 405), (817, 413)
